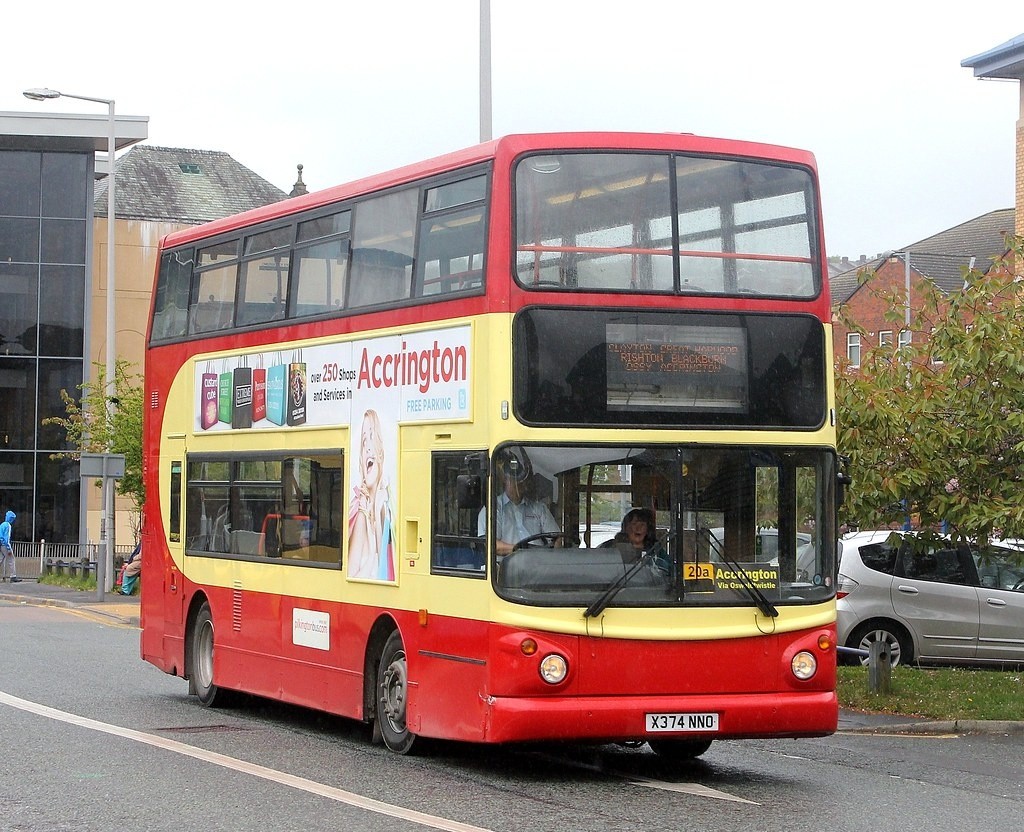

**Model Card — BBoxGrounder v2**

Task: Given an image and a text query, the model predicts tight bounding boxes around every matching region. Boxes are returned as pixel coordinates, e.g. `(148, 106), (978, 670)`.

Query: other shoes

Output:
(10, 577), (22, 583)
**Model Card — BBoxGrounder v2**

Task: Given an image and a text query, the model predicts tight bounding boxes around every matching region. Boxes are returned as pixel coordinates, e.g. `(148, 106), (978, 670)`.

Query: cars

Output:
(801, 531), (1024, 672)
(576, 520), (811, 578)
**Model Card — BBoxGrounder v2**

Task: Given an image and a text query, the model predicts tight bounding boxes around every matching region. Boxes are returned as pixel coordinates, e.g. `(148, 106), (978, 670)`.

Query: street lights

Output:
(22, 87), (116, 605)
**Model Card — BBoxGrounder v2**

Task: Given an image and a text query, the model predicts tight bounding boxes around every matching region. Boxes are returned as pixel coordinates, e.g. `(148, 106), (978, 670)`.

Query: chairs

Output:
(434, 543), (482, 570)
(209, 503), (254, 553)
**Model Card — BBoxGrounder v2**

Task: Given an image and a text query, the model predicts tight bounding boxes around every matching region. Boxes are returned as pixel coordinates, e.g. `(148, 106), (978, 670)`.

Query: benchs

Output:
(150, 302), (339, 339)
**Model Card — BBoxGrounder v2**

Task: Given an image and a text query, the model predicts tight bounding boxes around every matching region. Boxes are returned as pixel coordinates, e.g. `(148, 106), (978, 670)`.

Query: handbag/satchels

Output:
(232, 355), (252, 429)
(287, 349), (306, 427)
(265, 352), (287, 425)
(201, 361), (218, 430)
(219, 358), (232, 423)
(252, 353), (266, 422)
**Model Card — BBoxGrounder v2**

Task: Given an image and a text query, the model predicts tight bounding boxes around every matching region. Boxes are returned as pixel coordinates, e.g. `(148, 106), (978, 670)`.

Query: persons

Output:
(601, 509), (670, 567)
(117, 529), (142, 595)
(348, 408), (398, 583)
(0, 510), (22, 583)
(477, 459), (563, 566)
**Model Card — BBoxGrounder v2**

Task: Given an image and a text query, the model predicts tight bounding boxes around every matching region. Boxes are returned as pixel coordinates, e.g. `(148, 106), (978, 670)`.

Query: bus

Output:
(278, 452), (485, 570)
(138, 141), (852, 754)
